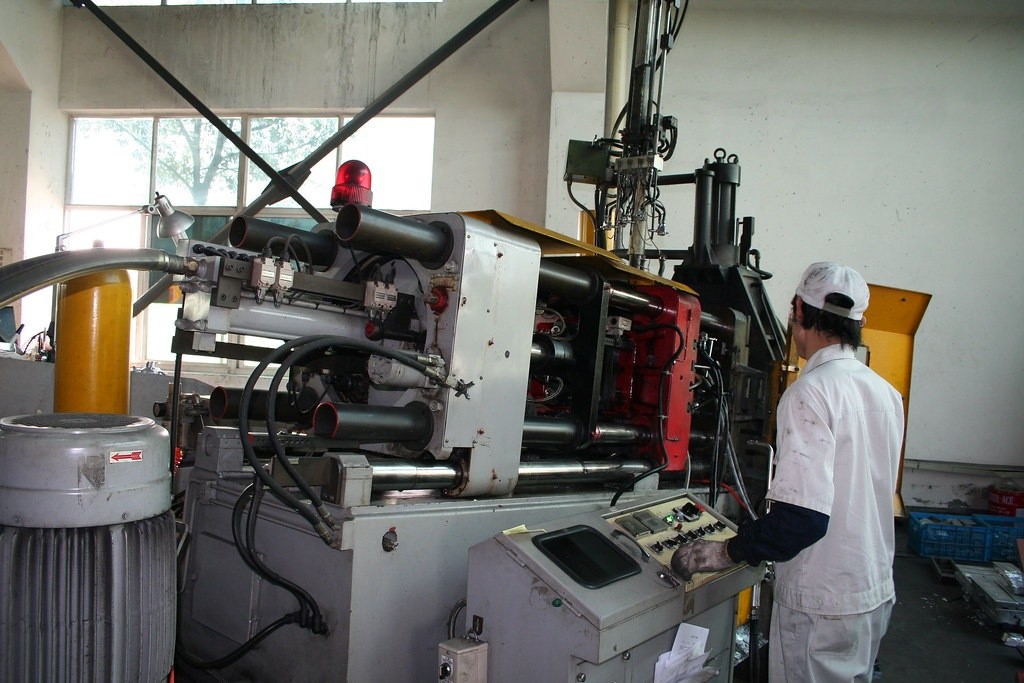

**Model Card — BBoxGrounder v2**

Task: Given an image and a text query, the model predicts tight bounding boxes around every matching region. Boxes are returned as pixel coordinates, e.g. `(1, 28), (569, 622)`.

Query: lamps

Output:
(48, 188), (195, 362)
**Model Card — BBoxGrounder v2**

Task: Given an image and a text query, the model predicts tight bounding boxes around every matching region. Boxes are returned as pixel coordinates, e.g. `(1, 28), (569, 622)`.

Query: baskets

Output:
(906, 511), (1024, 562)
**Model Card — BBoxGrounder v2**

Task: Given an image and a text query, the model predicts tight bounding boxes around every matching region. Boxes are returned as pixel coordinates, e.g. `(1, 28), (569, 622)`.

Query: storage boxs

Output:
(931, 557), (956, 588)
(909, 512), (990, 563)
(975, 514), (1024, 560)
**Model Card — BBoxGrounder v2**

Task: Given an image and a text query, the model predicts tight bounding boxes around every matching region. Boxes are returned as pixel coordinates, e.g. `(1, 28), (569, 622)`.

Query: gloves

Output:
(671, 539), (736, 581)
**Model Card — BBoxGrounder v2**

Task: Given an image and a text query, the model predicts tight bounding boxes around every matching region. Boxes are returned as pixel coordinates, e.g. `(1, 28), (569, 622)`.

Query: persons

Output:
(671, 262), (905, 683)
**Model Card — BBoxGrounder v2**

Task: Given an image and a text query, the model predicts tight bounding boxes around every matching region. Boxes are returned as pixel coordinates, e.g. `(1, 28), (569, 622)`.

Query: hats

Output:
(795, 262), (870, 321)
